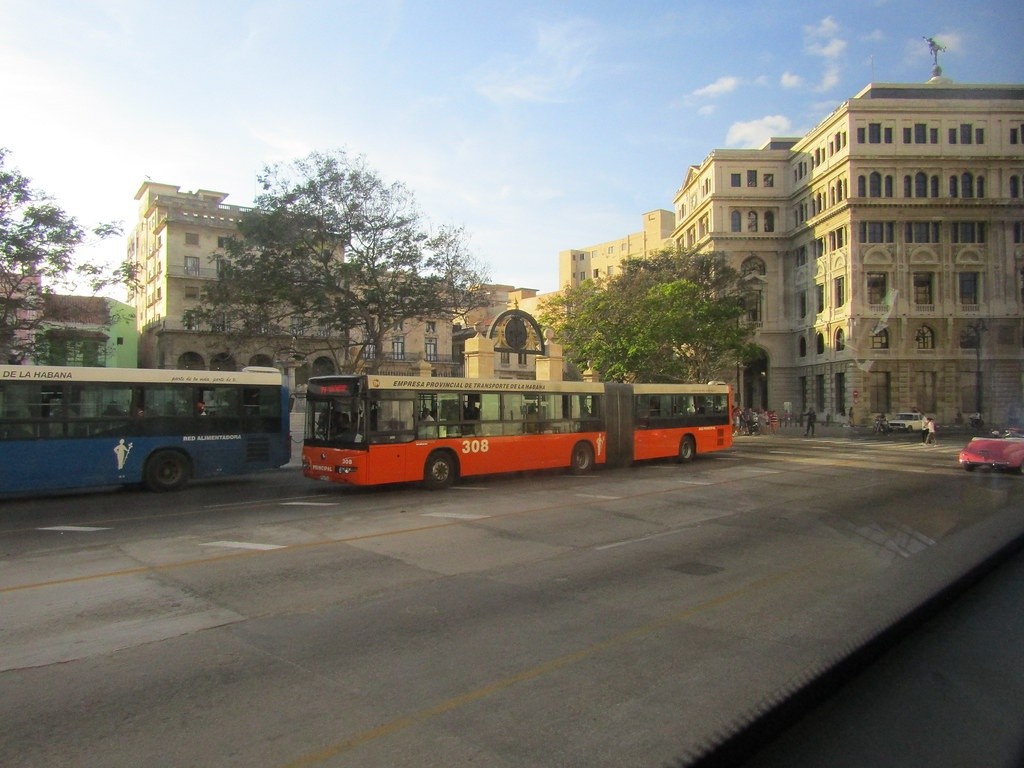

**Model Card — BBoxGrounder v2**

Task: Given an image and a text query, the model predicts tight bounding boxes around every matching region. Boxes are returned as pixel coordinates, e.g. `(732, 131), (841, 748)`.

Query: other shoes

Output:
(804, 434), (809, 436)
(812, 434), (816, 436)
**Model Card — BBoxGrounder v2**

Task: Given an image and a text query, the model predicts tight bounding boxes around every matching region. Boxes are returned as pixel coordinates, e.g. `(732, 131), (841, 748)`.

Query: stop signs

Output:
(853, 391), (859, 396)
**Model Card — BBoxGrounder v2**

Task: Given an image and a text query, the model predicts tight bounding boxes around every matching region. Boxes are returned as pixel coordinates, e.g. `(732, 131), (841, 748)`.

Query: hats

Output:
(420, 408), (430, 412)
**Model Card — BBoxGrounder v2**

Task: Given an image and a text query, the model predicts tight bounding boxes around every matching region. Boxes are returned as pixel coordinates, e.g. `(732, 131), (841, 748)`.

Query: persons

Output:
(920, 412), (939, 448)
(11, 400), (212, 437)
(847, 407), (856, 427)
(331, 404), (542, 441)
(973, 411), (980, 429)
(802, 406), (817, 436)
(731, 405), (779, 436)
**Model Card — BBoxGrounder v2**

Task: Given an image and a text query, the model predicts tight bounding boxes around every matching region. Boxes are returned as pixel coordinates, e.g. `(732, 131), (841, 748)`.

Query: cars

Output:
(959, 428), (1024, 476)
(889, 412), (922, 433)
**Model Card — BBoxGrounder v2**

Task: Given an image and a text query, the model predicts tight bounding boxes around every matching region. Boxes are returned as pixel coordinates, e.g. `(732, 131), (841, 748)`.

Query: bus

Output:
(0, 366), (291, 496)
(291, 374), (733, 490)
